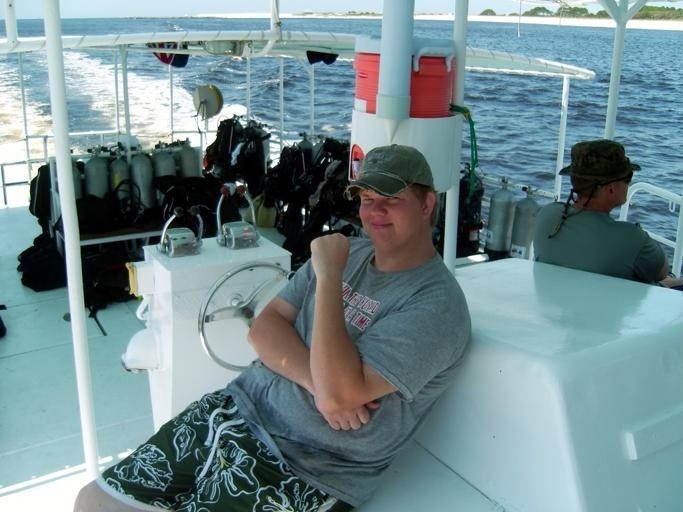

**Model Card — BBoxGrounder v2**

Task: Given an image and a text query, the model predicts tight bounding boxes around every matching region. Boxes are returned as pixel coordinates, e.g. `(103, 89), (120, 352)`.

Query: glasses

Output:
(602, 172), (633, 187)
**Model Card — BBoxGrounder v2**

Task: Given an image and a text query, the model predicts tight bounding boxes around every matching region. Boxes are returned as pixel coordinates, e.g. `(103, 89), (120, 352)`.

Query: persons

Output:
(532, 140), (682, 288)
(76, 144), (469, 509)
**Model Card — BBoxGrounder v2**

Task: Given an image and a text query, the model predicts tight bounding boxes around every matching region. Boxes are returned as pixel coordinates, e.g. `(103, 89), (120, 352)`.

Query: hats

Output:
(559, 139), (641, 181)
(346, 144), (433, 201)
